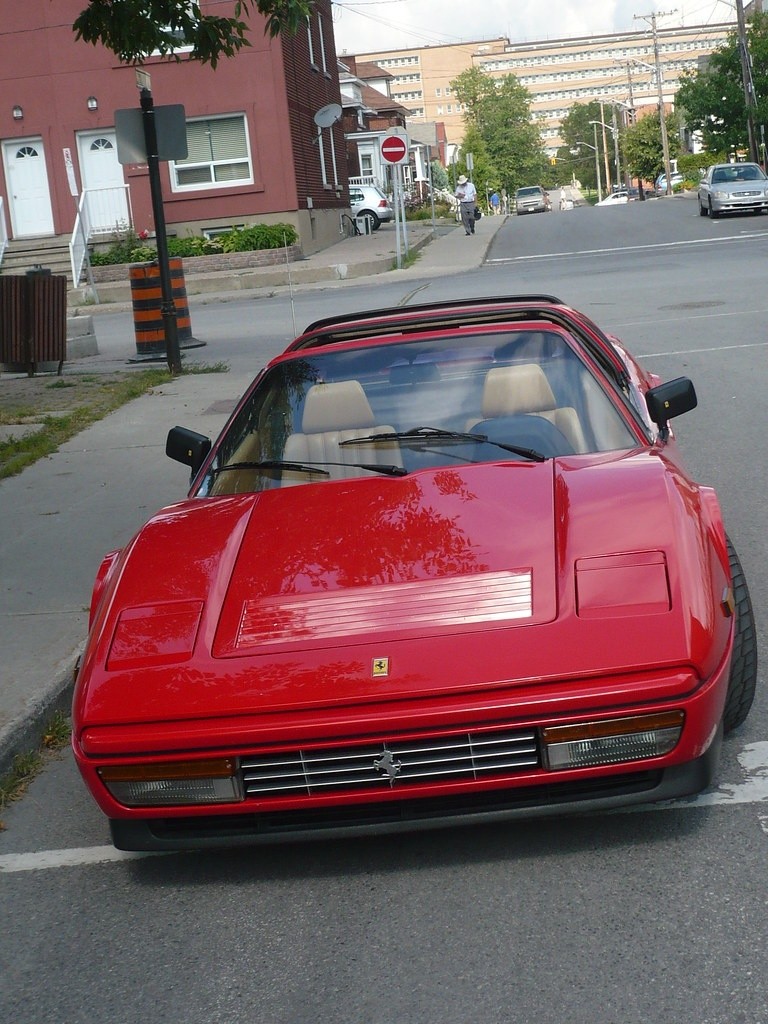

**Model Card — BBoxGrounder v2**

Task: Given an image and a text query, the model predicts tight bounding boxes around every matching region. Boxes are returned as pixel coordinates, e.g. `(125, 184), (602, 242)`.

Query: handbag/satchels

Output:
(474, 207), (482, 221)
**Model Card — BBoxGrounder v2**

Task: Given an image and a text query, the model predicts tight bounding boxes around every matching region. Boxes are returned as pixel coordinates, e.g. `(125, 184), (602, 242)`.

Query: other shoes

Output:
(471, 229), (476, 234)
(466, 232), (470, 235)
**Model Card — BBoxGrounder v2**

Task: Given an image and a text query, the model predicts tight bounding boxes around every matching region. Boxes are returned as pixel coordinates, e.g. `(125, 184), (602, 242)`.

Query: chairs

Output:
(466, 364), (587, 453)
(281, 380), (405, 487)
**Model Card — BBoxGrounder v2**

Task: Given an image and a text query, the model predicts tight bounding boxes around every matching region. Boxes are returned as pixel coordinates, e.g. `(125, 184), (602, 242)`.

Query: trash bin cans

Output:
(0, 274), (67, 371)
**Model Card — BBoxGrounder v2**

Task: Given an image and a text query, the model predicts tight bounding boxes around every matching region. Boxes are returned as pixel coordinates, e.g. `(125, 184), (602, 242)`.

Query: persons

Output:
(561, 187), (566, 202)
(490, 191), (500, 216)
(454, 175), (478, 235)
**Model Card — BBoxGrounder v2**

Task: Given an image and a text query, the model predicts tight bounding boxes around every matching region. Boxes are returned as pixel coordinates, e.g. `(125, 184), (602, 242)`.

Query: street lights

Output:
(574, 141), (601, 203)
(615, 55), (672, 195)
(588, 120), (622, 197)
(591, 97), (645, 201)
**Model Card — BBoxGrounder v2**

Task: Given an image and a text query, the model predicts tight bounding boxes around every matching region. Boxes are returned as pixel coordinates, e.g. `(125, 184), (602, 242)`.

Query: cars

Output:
(593, 191), (629, 207)
(697, 162), (768, 219)
(515, 184), (552, 215)
(349, 183), (395, 230)
(654, 172), (686, 196)
(627, 187), (647, 202)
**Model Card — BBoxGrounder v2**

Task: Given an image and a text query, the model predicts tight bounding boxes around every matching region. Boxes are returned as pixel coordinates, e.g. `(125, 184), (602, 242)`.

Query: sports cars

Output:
(66, 295), (760, 854)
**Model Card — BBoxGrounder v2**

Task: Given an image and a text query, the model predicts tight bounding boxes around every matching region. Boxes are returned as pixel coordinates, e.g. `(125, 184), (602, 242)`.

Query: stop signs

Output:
(381, 135), (407, 163)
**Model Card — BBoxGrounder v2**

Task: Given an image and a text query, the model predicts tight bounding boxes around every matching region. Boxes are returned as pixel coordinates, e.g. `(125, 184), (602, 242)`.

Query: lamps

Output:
(12, 105), (24, 120)
(87, 96), (98, 112)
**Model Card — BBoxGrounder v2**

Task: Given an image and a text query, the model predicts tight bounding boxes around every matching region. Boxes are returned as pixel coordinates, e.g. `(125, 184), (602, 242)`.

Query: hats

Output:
(457, 175), (468, 185)
